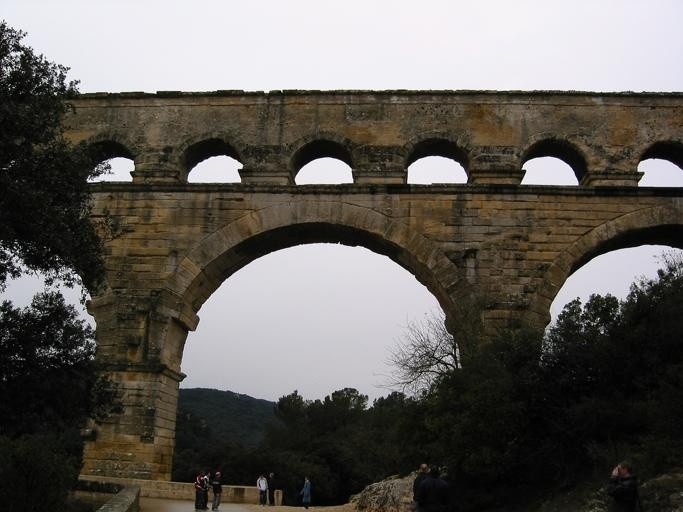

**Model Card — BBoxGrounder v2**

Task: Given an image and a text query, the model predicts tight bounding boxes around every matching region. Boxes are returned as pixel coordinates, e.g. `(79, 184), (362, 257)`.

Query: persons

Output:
(254, 471), (267, 506)
(298, 476), (312, 511)
(192, 468), (222, 512)
(265, 472), (276, 505)
(414, 464), (448, 512)
(411, 462), (429, 495)
(607, 459), (638, 510)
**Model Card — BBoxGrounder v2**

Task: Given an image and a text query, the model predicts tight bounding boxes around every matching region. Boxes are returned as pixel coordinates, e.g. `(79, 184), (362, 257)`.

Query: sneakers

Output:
(194, 506), (219, 512)
(258, 502), (275, 508)
